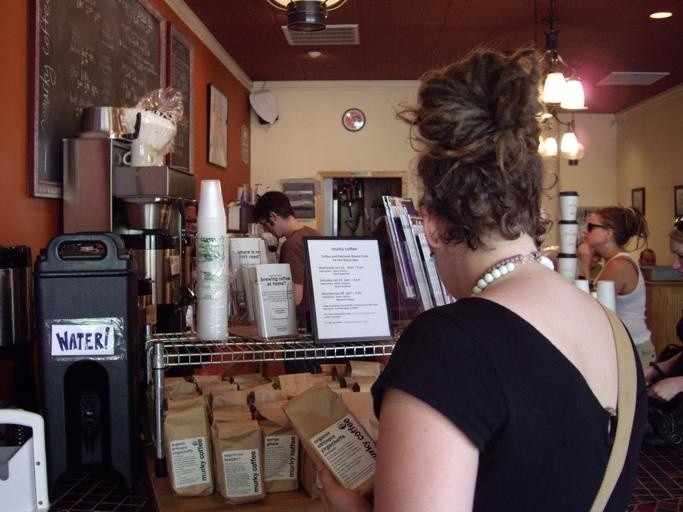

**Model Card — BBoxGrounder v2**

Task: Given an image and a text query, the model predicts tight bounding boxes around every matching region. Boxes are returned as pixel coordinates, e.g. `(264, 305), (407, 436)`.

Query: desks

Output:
(641, 267), (682, 351)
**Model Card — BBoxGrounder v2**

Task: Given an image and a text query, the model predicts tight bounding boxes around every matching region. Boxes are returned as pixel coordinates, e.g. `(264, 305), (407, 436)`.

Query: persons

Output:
(575, 202), (656, 388)
(638, 248), (658, 267)
(639, 218), (682, 450)
(317, 45), (649, 511)
(254, 192), (323, 330)
(575, 242), (603, 280)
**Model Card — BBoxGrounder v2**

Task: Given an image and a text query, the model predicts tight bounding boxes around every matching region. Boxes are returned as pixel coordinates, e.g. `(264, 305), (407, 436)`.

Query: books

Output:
(382, 196), (458, 311)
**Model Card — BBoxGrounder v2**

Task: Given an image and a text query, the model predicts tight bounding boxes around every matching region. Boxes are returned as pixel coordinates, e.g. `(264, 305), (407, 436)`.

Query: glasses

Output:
(673, 216), (683, 233)
(587, 222), (604, 233)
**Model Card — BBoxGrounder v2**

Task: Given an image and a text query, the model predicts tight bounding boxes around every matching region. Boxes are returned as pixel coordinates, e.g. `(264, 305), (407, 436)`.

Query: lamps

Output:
(534, 61), (589, 173)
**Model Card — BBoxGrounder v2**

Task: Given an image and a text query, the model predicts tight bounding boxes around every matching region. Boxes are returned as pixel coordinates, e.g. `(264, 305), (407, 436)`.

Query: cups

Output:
(119, 136), (167, 169)
(196, 179), (230, 343)
(555, 191), (617, 315)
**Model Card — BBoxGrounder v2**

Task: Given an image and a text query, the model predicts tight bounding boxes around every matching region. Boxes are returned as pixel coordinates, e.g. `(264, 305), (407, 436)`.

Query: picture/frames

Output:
(626, 184), (683, 218)
(202, 78), (232, 172)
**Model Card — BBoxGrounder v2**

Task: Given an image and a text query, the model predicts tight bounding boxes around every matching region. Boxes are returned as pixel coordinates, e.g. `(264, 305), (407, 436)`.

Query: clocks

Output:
(341, 104), (368, 133)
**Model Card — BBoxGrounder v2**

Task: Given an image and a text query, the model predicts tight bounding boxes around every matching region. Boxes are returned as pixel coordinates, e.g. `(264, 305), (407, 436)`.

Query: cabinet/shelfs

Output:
(140, 310), (397, 510)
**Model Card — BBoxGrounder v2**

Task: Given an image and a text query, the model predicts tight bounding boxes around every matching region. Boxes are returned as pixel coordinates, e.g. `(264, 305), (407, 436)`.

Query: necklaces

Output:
(466, 251), (555, 297)
